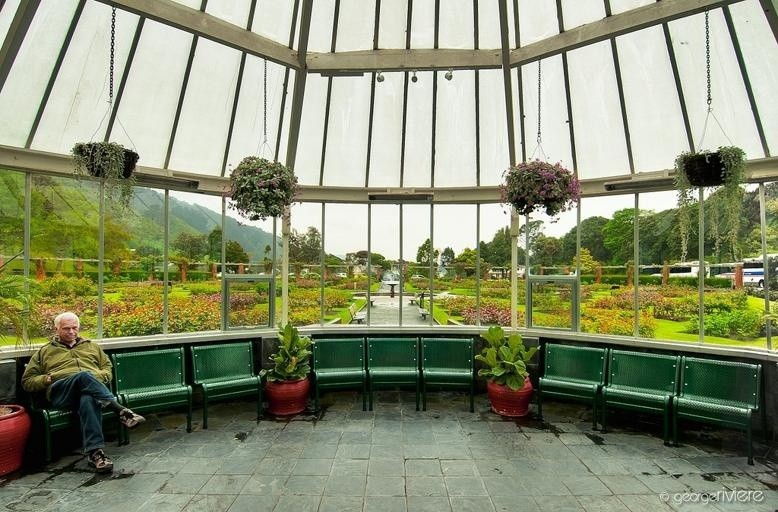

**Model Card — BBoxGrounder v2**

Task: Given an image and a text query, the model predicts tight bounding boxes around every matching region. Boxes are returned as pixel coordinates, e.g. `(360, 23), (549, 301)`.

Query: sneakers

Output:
(88, 449), (114, 472)
(119, 408), (146, 430)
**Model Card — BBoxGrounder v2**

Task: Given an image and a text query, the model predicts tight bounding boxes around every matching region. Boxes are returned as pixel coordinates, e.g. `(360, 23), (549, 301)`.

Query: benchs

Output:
(366, 293), (374, 306)
(348, 303), (367, 324)
(418, 303), (430, 320)
(408, 293), (418, 305)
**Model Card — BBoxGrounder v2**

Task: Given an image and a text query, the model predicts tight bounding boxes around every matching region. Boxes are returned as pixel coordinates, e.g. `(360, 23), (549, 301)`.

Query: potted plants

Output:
(69, 142), (139, 213)
(259, 324), (315, 415)
(673, 146), (747, 263)
(475, 326), (541, 417)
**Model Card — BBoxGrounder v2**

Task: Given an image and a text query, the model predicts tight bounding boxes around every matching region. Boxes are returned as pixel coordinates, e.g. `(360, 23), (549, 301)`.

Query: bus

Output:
(710, 258), (777, 290)
(649, 259), (713, 280)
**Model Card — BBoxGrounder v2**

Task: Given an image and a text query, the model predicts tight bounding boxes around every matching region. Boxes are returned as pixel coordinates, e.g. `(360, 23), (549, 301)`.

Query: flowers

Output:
(498, 161), (581, 216)
(227, 156), (298, 222)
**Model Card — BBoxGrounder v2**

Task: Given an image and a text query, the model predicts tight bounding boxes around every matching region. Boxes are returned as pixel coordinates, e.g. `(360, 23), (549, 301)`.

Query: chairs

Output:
(191, 342), (262, 429)
(114, 346), (193, 445)
(672, 355), (764, 465)
(26, 357), (124, 463)
(538, 342), (608, 430)
(310, 337), (367, 412)
(601, 347), (682, 445)
(420, 337), (474, 413)
(367, 336), (420, 411)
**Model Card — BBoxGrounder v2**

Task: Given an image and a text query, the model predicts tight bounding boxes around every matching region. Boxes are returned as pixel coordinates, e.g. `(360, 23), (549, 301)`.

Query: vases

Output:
(0, 405), (31, 476)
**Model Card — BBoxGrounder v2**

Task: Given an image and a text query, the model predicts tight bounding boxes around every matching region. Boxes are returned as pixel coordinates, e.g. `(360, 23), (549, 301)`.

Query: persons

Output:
(21, 312), (146, 473)
